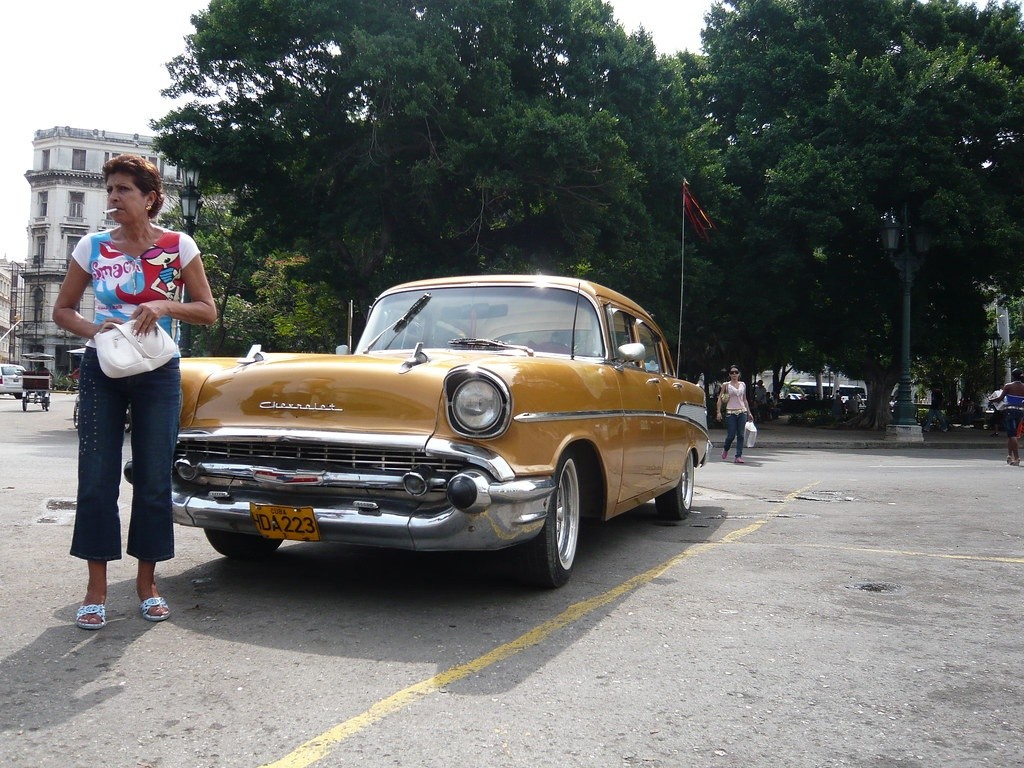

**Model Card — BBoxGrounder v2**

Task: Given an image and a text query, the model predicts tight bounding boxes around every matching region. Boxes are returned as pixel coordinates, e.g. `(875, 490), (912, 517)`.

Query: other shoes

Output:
(924, 428), (929, 432)
(734, 457), (744, 463)
(943, 427), (948, 432)
(721, 449), (728, 459)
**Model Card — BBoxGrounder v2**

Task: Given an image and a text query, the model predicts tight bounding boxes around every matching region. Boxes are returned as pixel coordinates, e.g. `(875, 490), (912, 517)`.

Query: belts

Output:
(1005, 406), (1023, 410)
(726, 408), (747, 415)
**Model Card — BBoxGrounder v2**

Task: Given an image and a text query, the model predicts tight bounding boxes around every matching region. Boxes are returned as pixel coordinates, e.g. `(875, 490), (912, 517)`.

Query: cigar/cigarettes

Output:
(103, 208), (118, 214)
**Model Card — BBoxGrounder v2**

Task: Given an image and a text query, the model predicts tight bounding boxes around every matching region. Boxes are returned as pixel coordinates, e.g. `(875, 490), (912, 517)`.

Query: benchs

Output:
(22, 371), (52, 391)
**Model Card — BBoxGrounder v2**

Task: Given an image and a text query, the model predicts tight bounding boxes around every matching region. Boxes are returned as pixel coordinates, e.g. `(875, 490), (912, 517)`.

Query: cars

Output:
(0, 364), (26, 399)
(123, 274), (713, 590)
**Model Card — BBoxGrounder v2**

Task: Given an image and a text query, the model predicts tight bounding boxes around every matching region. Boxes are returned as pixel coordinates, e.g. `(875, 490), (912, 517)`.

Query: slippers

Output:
(76, 604), (107, 630)
(140, 597), (172, 621)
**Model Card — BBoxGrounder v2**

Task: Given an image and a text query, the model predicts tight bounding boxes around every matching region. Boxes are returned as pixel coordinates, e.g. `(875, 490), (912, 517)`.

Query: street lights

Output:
(886, 187), (934, 425)
(989, 328), (1002, 429)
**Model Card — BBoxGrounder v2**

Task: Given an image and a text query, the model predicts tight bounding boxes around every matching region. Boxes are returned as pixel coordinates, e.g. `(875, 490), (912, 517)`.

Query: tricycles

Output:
(20, 352), (55, 412)
(65, 347), (132, 433)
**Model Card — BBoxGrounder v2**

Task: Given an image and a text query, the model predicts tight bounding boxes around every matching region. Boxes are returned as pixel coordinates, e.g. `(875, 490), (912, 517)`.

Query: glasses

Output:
(730, 371), (739, 374)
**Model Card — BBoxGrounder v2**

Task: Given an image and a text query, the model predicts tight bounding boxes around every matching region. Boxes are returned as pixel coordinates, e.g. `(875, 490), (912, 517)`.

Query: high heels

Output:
(1010, 458), (1020, 466)
(1006, 455), (1012, 464)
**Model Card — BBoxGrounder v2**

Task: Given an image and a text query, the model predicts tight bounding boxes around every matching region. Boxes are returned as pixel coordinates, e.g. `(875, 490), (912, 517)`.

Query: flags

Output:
(683, 186), (719, 242)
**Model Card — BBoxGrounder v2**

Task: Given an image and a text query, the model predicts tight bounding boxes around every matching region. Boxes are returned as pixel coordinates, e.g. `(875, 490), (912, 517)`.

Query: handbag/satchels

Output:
(744, 420), (757, 447)
(93, 317), (175, 378)
(721, 382), (729, 403)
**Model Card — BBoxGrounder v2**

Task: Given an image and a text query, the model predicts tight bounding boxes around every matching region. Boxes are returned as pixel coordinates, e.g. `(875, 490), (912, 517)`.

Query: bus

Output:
(778, 382), (866, 412)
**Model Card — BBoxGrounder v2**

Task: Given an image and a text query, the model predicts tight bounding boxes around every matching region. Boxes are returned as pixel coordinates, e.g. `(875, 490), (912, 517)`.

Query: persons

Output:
(34, 362), (50, 374)
(751, 380), (779, 425)
(716, 365), (754, 463)
(51, 152), (217, 630)
(831, 390), (862, 418)
(923, 383), (948, 433)
(988, 368), (1024, 466)
